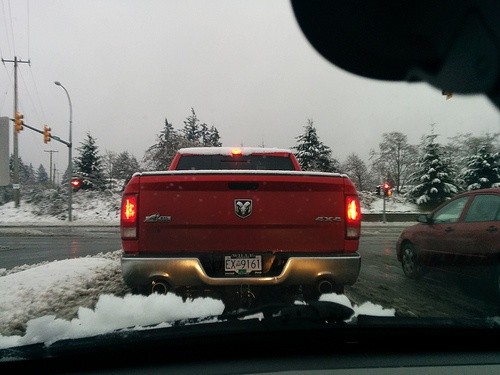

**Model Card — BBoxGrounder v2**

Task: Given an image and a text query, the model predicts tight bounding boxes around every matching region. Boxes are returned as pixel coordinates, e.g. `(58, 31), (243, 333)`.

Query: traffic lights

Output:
(44, 124), (51, 144)
(71, 179), (80, 193)
(15, 111), (24, 134)
(375, 186), (381, 196)
(384, 184), (389, 197)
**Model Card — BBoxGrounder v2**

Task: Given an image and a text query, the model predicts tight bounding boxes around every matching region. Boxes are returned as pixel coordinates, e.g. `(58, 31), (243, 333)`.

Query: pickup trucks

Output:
(120, 146), (362, 307)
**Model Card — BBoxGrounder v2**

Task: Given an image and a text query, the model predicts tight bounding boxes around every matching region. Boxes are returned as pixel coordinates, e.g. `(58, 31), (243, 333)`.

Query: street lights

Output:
(54, 80), (73, 222)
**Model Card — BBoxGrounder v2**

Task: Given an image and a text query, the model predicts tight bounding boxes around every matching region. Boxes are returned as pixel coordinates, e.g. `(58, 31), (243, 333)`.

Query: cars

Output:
(395, 188), (500, 287)
